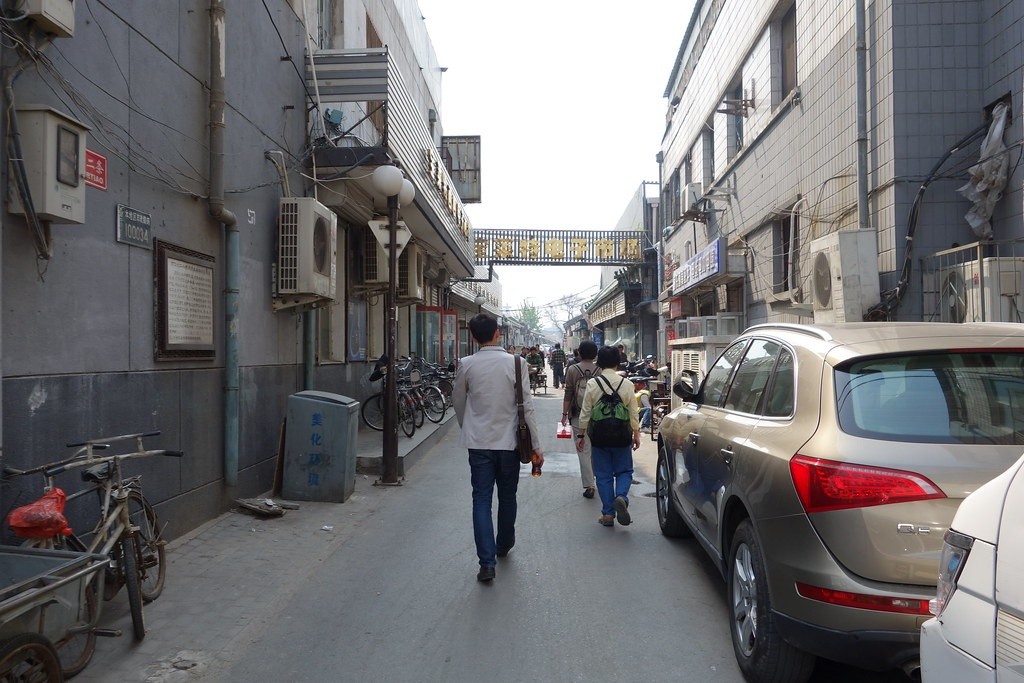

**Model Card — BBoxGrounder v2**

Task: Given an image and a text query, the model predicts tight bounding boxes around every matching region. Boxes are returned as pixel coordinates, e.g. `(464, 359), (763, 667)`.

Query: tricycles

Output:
(0, 442), (153, 683)
(528, 363), (547, 396)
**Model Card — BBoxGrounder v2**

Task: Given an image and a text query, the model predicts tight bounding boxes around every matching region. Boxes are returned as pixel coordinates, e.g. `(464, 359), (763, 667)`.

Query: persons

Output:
(452, 313), (545, 581)
(634, 380), (661, 434)
(576, 346), (640, 526)
(519, 344), (545, 388)
(548, 344), (581, 389)
(561, 341), (602, 497)
(509, 346), (516, 355)
(618, 346), (628, 371)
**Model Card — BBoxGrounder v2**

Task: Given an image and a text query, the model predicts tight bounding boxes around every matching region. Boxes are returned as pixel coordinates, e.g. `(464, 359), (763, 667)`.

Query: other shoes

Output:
(477, 567), (495, 581)
(497, 545), (508, 558)
(639, 427), (657, 433)
(583, 489), (594, 498)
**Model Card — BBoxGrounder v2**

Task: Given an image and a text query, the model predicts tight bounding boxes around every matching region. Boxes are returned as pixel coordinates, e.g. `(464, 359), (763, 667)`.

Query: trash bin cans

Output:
(281, 390), (361, 504)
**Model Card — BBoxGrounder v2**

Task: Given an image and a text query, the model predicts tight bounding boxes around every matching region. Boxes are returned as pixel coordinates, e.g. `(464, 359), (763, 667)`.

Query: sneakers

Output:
(615, 497), (630, 526)
(599, 515), (614, 526)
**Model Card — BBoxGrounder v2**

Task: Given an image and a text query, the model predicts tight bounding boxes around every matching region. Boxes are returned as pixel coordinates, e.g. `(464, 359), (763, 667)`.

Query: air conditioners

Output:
(808, 224), (881, 321)
(271, 192), (343, 301)
(939, 257), (1024, 323)
(679, 181), (701, 218)
(397, 245), (429, 300)
(362, 213), (400, 288)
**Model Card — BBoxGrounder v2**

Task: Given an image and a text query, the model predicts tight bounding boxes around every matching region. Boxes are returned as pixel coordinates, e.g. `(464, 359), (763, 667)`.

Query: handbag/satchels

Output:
(517, 424), (532, 463)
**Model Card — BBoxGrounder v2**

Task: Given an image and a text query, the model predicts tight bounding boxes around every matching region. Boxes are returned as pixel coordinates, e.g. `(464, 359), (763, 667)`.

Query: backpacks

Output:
(586, 375), (632, 448)
(572, 364), (600, 417)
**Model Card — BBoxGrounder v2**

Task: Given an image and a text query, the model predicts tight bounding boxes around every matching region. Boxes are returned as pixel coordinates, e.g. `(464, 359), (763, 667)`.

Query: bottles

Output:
(531, 451), (541, 478)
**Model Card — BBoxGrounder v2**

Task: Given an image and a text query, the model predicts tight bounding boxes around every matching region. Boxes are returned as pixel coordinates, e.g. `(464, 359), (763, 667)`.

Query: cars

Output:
(916, 448), (1024, 683)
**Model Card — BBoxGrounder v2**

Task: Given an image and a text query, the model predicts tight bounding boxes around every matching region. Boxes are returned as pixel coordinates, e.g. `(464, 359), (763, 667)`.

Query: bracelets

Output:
(576, 433), (585, 438)
(562, 413), (567, 415)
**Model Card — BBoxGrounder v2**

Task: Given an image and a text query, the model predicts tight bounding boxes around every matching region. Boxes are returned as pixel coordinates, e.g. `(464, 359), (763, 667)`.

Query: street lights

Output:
(371, 164), (415, 486)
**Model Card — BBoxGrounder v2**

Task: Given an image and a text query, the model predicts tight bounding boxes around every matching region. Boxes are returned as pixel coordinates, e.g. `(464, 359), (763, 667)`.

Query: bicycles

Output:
(0, 425), (169, 602)
(361, 354), (462, 438)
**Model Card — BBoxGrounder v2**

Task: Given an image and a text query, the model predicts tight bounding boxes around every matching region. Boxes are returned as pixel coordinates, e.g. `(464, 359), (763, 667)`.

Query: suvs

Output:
(654, 321), (1024, 683)
